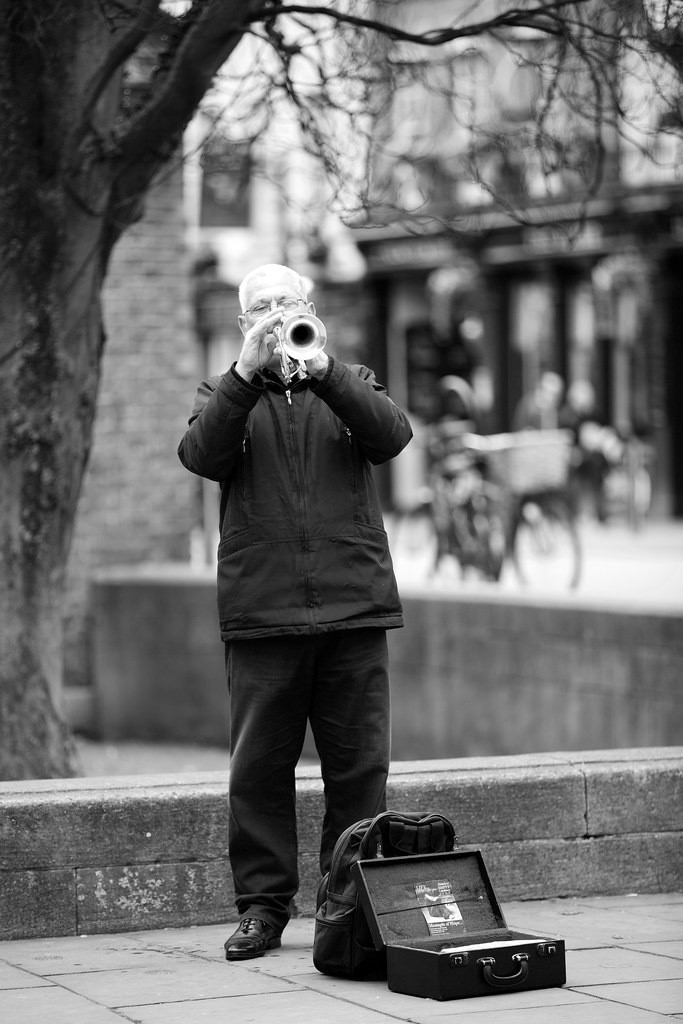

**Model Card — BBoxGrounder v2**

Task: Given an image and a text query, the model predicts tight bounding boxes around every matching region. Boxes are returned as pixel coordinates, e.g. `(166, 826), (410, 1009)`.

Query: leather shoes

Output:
(223, 918), (281, 960)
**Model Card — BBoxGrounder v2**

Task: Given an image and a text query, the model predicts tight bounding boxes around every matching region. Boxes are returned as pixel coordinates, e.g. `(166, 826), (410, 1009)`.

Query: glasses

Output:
(243, 297), (308, 317)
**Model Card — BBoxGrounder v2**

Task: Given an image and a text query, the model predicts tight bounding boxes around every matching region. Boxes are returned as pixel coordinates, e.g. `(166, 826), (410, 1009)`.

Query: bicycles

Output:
(425, 418), (580, 595)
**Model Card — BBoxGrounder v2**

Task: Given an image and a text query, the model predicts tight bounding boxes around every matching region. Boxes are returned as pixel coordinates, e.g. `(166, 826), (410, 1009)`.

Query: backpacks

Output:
(314, 811), (455, 980)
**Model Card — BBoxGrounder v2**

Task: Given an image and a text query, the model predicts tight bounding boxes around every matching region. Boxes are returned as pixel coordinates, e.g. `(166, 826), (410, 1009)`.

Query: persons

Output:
(392, 372), (616, 531)
(176, 264), (413, 960)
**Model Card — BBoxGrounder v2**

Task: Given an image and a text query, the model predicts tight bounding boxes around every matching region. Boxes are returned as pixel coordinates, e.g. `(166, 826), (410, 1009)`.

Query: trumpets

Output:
(273, 312), (328, 383)
